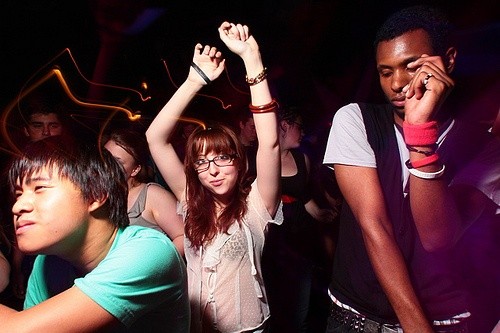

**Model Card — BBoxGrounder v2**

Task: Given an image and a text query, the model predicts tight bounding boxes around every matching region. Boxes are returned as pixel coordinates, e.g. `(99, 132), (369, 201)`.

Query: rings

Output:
(424, 73), (433, 85)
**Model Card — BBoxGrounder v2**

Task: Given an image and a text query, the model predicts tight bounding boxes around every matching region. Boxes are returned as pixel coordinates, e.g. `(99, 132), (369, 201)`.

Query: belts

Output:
(328, 301), (403, 332)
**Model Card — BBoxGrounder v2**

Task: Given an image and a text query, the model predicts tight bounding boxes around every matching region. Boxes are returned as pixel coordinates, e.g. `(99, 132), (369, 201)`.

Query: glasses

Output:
(191, 153), (234, 169)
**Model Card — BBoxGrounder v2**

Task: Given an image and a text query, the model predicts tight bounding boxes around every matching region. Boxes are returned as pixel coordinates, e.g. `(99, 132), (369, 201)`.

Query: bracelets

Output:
(191, 63), (211, 84)
(402, 122), (445, 179)
(246, 67), (267, 86)
(249, 99), (279, 113)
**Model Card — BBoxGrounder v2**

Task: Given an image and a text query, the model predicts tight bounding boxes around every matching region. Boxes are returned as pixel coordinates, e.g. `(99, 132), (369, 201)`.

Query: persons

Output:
(103, 129), (184, 258)
(0, 132), (191, 333)
(9, 105), (67, 300)
(235, 107), (342, 333)
(144, 19), (283, 333)
(321, 5), (500, 333)
